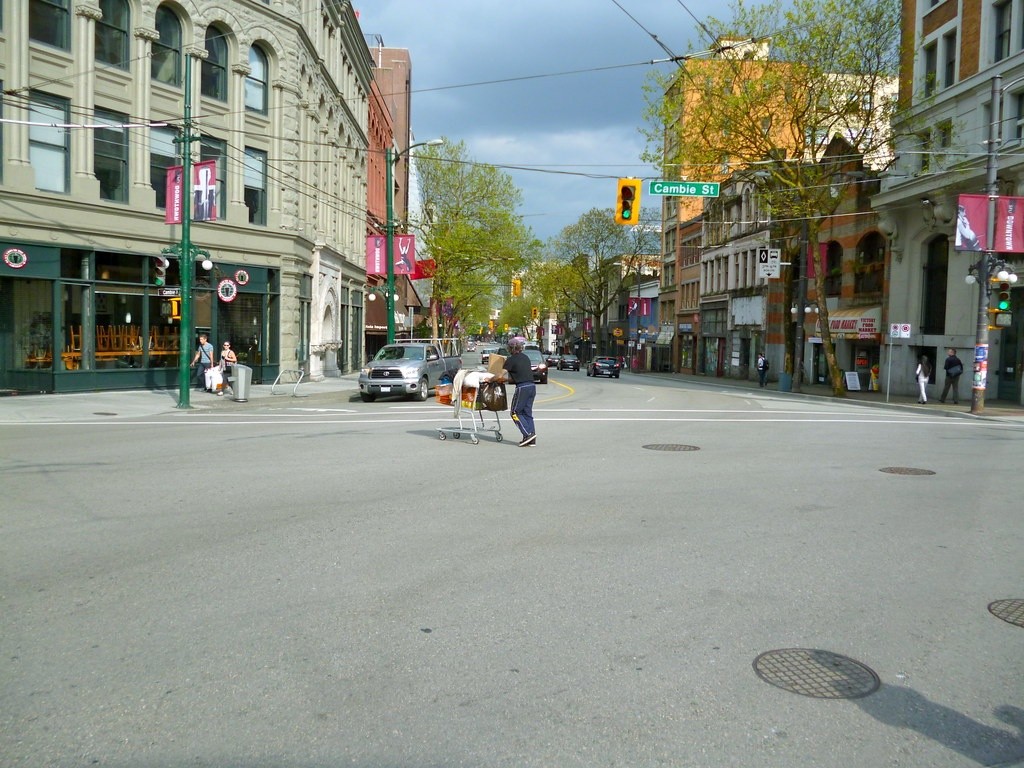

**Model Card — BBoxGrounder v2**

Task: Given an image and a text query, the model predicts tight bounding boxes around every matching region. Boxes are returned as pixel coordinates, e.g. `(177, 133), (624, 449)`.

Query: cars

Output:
(543, 354), (580, 372)
(481, 348), (497, 365)
(587, 356), (620, 378)
(467, 343), (476, 351)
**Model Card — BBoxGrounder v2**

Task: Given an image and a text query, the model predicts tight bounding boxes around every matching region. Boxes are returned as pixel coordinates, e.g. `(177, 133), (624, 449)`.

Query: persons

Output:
(916, 355), (932, 404)
(756, 352), (767, 387)
(190, 334), (213, 391)
(484, 338), (537, 447)
(218, 341), (237, 396)
(937, 348), (963, 404)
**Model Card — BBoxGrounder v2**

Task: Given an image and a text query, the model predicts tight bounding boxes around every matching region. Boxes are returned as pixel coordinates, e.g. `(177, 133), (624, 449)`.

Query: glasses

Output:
(224, 345), (229, 346)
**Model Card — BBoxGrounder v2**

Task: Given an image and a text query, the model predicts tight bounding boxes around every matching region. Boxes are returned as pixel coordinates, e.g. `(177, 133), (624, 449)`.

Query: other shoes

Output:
(519, 433), (537, 447)
(218, 391), (223, 395)
(528, 439), (536, 447)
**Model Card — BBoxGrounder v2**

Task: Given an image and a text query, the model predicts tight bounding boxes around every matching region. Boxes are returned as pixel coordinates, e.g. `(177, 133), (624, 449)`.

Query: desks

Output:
(27, 349), (181, 370)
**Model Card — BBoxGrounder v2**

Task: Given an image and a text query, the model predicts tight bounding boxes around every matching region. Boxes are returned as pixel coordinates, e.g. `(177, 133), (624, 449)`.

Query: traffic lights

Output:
(616, 178), (641, 225)
(153, 256), (165, 287)
(532, 307), (539, 318)
(995, 311), (1013, 327)
(159, 299), (177, 318)
(999, 281), (1011, 312)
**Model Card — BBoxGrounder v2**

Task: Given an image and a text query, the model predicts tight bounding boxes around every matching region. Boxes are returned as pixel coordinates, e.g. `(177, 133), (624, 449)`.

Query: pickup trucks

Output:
(357, 343), (463, 402)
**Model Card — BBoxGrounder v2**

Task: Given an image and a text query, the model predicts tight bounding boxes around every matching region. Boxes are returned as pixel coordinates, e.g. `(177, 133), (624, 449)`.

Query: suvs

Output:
(498, 344), (548, 385)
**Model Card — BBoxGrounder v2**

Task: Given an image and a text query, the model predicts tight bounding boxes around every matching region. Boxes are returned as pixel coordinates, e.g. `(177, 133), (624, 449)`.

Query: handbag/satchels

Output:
(211, 372), (223, 392)
(947, 365), (962, 378)
(226, 366), (231, 373)
(915, 374), (918, 383)
(480, 383), (508, 411)
(204, 367), (218, 389)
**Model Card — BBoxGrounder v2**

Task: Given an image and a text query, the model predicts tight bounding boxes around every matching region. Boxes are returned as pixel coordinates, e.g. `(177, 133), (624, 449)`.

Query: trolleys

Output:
(434, 377), (503, 445)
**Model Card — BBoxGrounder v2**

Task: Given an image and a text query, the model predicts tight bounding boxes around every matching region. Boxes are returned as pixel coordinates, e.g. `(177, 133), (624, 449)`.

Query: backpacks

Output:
(760, 357), (769, 371)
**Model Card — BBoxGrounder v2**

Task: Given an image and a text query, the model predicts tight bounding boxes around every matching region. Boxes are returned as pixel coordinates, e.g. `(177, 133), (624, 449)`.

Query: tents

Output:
(815, 305), (882, 333)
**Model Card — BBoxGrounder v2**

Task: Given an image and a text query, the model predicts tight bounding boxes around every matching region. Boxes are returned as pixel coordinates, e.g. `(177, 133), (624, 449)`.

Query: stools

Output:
(28, 325), (178, 359)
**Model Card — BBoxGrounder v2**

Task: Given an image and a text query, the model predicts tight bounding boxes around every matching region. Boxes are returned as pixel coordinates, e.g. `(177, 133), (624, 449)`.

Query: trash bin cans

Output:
(778, 371), (791, 391)
(228, 364), (252, 402)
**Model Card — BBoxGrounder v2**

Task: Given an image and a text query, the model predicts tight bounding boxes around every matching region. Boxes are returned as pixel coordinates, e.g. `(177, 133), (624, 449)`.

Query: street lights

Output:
(613, 262), (641, 343)
(385, 139), (444, 343)
(754, 170), (864, 394)
